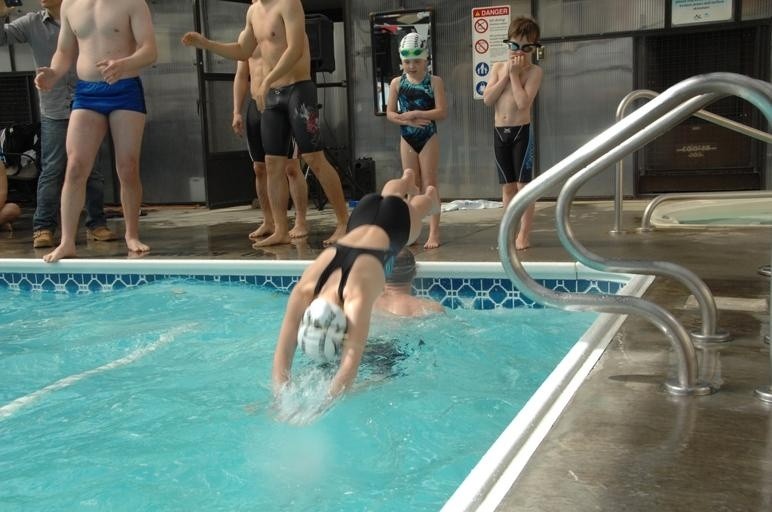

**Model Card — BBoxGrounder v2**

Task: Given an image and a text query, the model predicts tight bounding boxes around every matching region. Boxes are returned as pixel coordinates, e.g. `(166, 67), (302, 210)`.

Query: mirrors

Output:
(368, 7), (437, 116)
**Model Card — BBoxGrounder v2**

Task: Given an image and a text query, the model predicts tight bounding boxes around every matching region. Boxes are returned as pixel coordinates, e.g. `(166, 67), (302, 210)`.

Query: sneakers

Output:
(86, 226), (118, 241)
(33, 230), (53, 249)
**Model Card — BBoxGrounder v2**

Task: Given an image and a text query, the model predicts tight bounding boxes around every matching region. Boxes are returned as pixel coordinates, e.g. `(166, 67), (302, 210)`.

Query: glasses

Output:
(401, 48), (425, 56)
(503, 38), (540, 53)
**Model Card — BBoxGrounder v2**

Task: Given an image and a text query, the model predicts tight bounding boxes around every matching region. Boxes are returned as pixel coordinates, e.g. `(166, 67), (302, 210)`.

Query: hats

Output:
(384, 248), (417, 285)
(399, 32), (429, 60)
(297, 298), (348, 367)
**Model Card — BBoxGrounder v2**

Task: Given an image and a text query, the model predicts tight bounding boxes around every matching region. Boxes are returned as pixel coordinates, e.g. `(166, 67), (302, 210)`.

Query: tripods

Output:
(287, 72), (366, 211)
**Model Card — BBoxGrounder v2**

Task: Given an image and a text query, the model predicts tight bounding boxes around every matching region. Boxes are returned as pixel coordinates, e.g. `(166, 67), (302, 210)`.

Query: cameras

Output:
(5, 0), (22, 7)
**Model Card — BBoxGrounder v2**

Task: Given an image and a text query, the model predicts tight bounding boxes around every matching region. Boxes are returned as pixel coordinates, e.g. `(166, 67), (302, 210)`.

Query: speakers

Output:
(351, 159), (375, 201)
(305, 14), (335, 73)
(373, 32), (407, 81)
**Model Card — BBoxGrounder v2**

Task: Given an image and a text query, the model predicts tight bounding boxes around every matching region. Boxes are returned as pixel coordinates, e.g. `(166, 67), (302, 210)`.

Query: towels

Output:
(440, 198), (503, 212)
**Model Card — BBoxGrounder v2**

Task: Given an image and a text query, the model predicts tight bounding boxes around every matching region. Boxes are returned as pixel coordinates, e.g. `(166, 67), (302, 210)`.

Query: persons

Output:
(180, 0), (351, 249)
(272, 168), (441, 425)
(0, 0), (122, 249)
(232, 0), (312, 238)
(482, 16), (543, 250)
(0, 159), (22, 240)
(32, 0), (158, 264)
(386, 32), (448, 249)
(371, 246), (447, 318)
